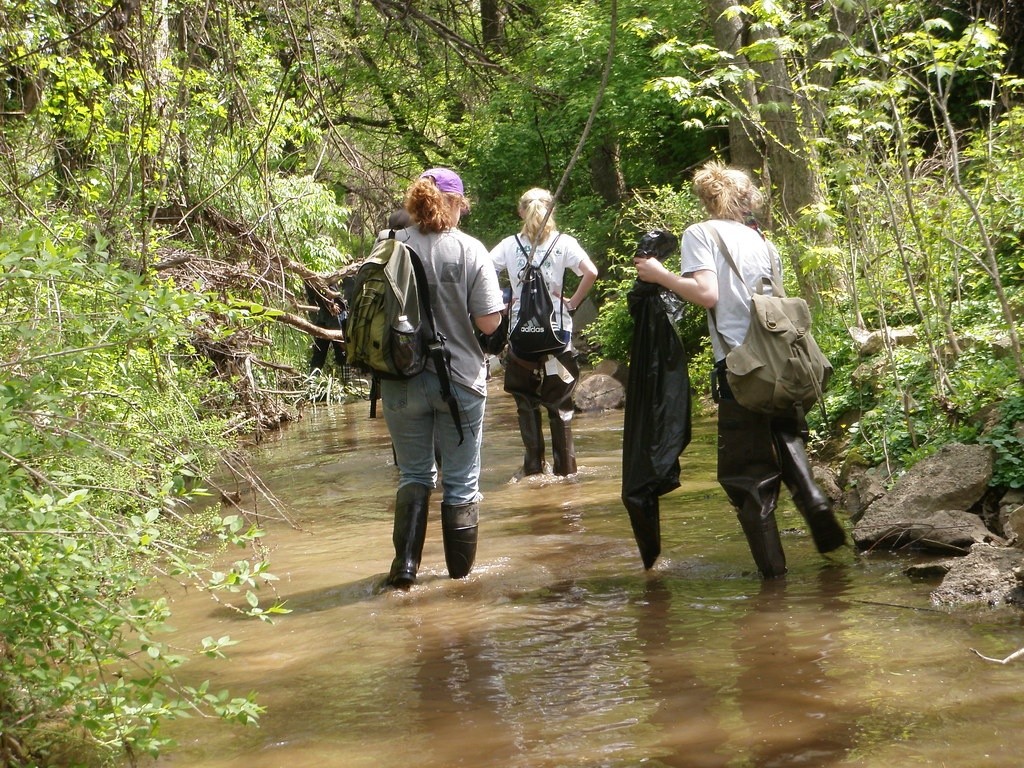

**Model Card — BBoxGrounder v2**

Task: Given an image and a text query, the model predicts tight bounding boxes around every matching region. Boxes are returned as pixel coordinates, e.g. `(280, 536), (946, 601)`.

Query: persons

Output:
(490, 187), (598, 475)
(379, 169), (506, 589)
(634, 165), (846, 581)
(303, 260), (364, 374)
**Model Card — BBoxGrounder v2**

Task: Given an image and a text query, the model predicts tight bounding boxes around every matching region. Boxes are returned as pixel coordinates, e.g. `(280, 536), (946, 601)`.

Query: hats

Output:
(420, 168), (468, 213)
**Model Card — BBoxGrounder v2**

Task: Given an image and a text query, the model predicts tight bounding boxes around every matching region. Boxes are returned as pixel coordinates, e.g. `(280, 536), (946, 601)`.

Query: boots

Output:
(780, 439), (846, 555)
(388, 483), (430, 590)
(550, 422), (577, 476)
(517, 408), (545, 476)
(441, 502), (478, 580)
(737, 504), (787, 585)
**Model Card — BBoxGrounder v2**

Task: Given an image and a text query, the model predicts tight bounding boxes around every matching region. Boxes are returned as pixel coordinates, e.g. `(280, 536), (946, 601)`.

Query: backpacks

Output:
(697, 220), (831, 415)
(509, 232), (573, 361)
(346, 230), (476, 447)
